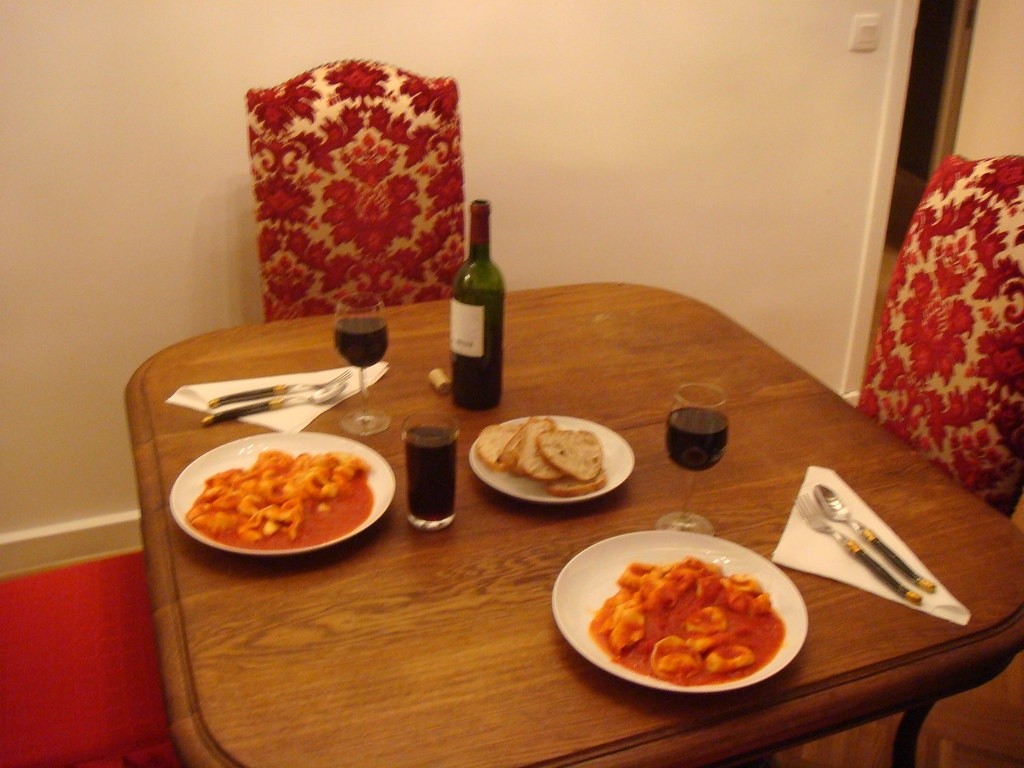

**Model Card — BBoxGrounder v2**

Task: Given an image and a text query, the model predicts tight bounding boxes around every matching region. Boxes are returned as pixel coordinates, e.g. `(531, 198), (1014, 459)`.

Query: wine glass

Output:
(332, 291), (393, 436)
(653, 382), (730, 537)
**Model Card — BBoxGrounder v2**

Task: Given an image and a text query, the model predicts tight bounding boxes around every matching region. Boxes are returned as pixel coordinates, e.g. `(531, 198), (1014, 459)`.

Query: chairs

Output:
(246, 59), (466, 325)
(1, 549), (181, 768)
(857, 154), (1024, 519)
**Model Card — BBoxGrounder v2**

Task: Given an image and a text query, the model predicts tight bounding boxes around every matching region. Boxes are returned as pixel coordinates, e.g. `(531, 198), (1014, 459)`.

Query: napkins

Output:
(772, 464), (971, 626)
(166, 361), (389, 433)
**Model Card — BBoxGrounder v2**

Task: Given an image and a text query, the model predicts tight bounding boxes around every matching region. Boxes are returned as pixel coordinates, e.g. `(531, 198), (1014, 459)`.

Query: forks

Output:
(791, 494), (922, 605)
(208, 365), (353, 408)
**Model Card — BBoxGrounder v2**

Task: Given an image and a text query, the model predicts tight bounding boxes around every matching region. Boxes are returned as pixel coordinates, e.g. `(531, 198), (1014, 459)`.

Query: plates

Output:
(552, 530), (809, 695)
(170, 432), (397, 557)
(468, 414), (636, 502)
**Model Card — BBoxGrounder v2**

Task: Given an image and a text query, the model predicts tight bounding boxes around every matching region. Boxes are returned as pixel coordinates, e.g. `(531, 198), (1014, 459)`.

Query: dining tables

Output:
(127, 282), (1024, 768)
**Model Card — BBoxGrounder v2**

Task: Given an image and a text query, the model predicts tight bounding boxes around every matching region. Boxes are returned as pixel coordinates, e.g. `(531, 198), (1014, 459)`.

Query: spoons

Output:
(201, 381), (347, 426)
(813, 484), (935, 593)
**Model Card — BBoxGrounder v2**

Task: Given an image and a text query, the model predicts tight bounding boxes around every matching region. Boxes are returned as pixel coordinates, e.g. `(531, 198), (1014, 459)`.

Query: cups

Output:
(400, 412), (462, 532)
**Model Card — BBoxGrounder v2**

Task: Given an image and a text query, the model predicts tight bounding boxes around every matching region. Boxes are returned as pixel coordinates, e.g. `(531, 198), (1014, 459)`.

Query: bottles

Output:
(449, 198), (506, 411)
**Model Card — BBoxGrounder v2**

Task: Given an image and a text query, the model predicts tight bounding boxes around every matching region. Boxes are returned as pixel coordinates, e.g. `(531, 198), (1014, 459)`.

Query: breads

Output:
(474, 418), (607, 498)
